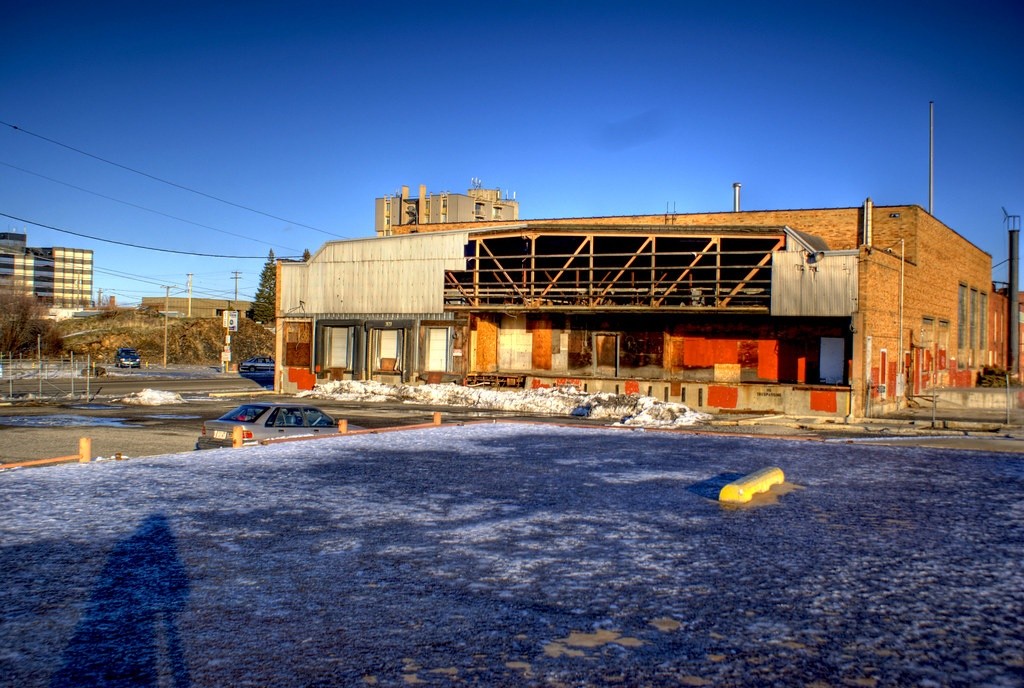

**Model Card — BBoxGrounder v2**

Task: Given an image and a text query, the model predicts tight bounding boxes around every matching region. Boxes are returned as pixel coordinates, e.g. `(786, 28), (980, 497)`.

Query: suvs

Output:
(115, 347), (142, 368)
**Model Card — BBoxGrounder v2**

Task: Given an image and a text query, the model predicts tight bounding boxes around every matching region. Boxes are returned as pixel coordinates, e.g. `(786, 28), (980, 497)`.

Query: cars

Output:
(239, 354), (275, 372)
(194, 401), (366, 450)
